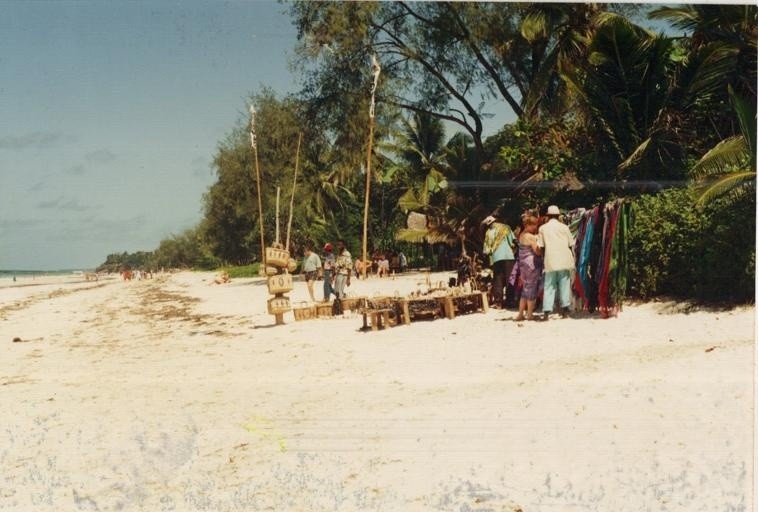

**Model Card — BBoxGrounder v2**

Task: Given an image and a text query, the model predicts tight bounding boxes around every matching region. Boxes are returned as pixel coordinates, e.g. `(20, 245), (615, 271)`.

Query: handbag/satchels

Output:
(293, 280), (405, 322)
(267, 267), (294, 294)
(268, 297), (291, 314)
(265, 242), (290, 267)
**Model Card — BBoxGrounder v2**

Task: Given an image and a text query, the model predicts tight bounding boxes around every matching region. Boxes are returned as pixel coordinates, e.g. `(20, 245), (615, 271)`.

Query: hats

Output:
(547, 205), (560, 215)
(486, 216), (496, 226)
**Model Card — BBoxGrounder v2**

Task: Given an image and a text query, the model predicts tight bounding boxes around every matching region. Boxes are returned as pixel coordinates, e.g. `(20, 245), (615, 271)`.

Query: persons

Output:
(536, 205), (576, 321)
(317, 243), (346, 303)
(301, 241), (322, 302)
(517, 211), (544, 321)
(330, 239), (352, 302)
(481, 215), (520, 308)
(120, 265), (165, 282)
(356, 246), (410, 280)
